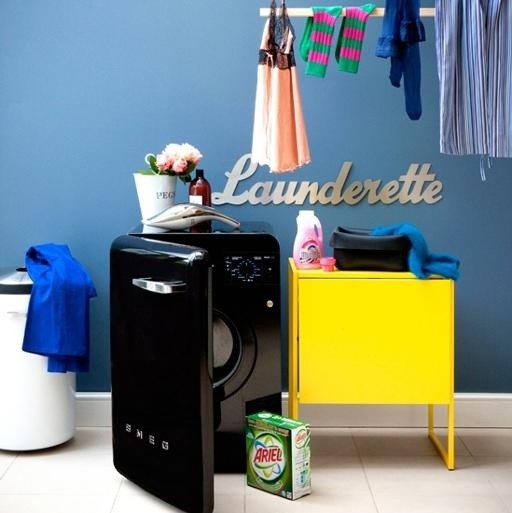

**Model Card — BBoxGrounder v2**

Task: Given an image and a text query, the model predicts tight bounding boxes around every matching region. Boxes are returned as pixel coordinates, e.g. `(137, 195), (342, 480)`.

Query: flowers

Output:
(143, 138), (202, 183)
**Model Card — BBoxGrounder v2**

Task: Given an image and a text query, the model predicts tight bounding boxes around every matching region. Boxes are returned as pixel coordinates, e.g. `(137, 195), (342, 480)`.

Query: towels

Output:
(21, 243), (98, 374)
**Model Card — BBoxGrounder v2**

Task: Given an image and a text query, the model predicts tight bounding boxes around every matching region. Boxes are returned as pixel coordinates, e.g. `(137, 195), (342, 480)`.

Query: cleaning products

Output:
(292, 209), (326, 270)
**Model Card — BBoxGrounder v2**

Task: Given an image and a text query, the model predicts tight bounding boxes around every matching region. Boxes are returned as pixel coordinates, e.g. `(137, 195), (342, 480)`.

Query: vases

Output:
(132, 172), (179, 224)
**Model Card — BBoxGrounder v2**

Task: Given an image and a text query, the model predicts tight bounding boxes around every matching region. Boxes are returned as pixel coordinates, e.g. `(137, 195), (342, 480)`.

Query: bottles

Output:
(188, 169), (212, 227)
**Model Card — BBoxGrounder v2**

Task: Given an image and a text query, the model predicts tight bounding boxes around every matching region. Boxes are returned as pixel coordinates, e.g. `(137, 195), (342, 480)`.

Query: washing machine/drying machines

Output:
(108, 219), (284, 512)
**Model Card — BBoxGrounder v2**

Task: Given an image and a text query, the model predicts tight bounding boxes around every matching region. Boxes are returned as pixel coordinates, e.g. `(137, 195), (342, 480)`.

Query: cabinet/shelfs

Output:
(286, 256), (456, 474)
